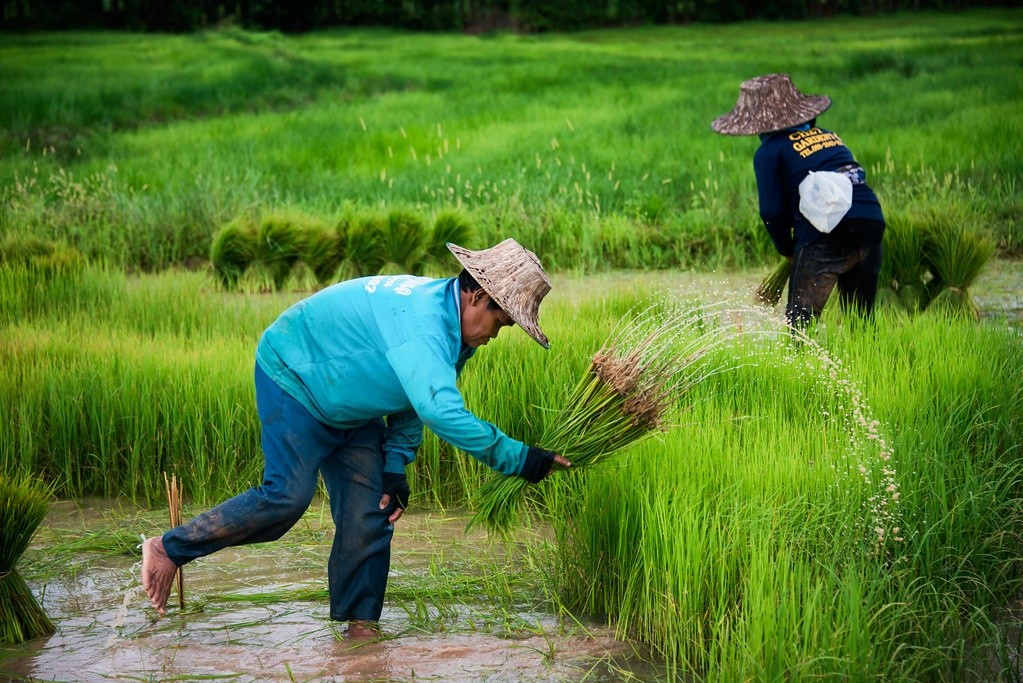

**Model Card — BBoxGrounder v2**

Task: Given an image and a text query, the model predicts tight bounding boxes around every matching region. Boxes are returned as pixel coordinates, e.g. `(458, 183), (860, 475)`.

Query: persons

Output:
(141, 238), (571, 636)
(711, 73), (886, 350)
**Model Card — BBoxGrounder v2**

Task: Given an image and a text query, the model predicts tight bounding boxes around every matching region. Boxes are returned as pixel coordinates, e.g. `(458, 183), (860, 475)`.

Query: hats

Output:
(709, 74), (830, 135)
(444, 238), (552, 349)
